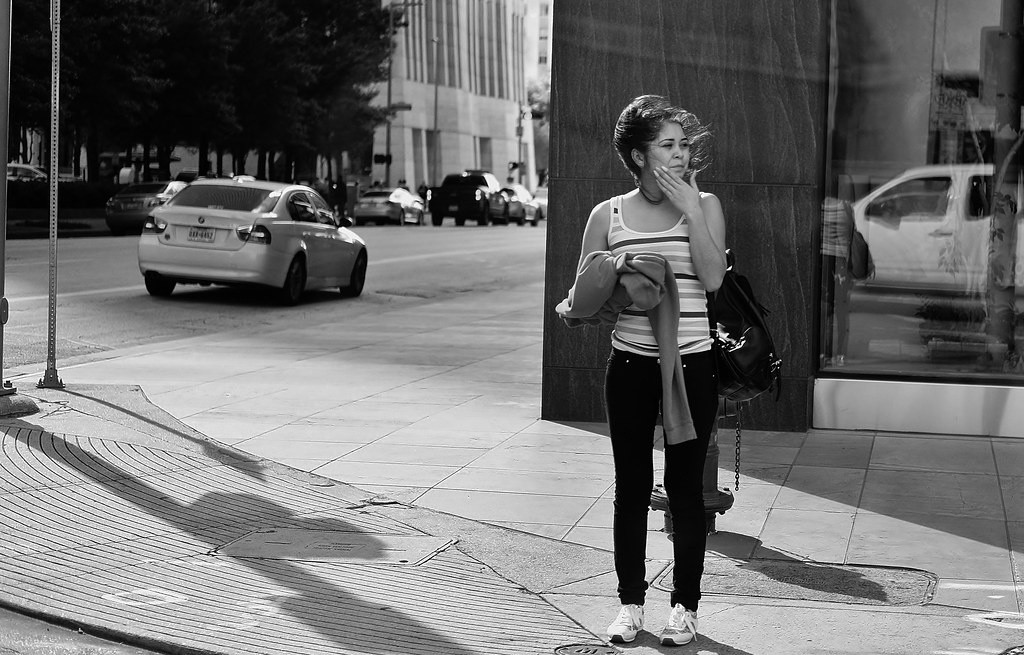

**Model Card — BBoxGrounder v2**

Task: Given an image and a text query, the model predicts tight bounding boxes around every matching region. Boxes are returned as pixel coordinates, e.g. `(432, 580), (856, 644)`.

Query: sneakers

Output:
(659, 603), (698, 647)
(607, 603), (645, 644)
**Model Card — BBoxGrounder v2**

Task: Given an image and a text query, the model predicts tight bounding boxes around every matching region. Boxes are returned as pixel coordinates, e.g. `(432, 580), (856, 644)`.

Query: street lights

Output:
(516, 112), (534, 182)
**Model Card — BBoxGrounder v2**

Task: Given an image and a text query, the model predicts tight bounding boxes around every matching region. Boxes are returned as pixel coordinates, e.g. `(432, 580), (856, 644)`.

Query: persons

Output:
(417, 182), (428, 212)
(821, 194), (855, 368)
(555, 94), (728, 646)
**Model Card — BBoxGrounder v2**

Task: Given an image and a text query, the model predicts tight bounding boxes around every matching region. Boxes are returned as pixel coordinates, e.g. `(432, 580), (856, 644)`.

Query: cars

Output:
(850, 163), (1024, 296)
(6, 163), (50, 183)
(136, 173), (369, 306)
(500, 183), (548, 227)
(353, 186), (425, 226)
(104, 179), (189, 237)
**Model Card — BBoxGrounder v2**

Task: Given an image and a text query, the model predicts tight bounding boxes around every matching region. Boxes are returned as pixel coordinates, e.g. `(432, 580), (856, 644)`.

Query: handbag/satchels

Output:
(847, 221), (876, 282)
(706, 247), (783, 404)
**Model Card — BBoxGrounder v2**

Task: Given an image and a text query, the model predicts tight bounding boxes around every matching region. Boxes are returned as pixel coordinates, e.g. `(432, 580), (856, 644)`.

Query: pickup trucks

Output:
(430, 167), (515, 228)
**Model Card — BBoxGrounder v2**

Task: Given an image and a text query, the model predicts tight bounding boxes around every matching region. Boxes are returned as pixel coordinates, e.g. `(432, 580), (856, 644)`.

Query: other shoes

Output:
(819, 354), (833, 368)
(833, 353), (849, 367)
